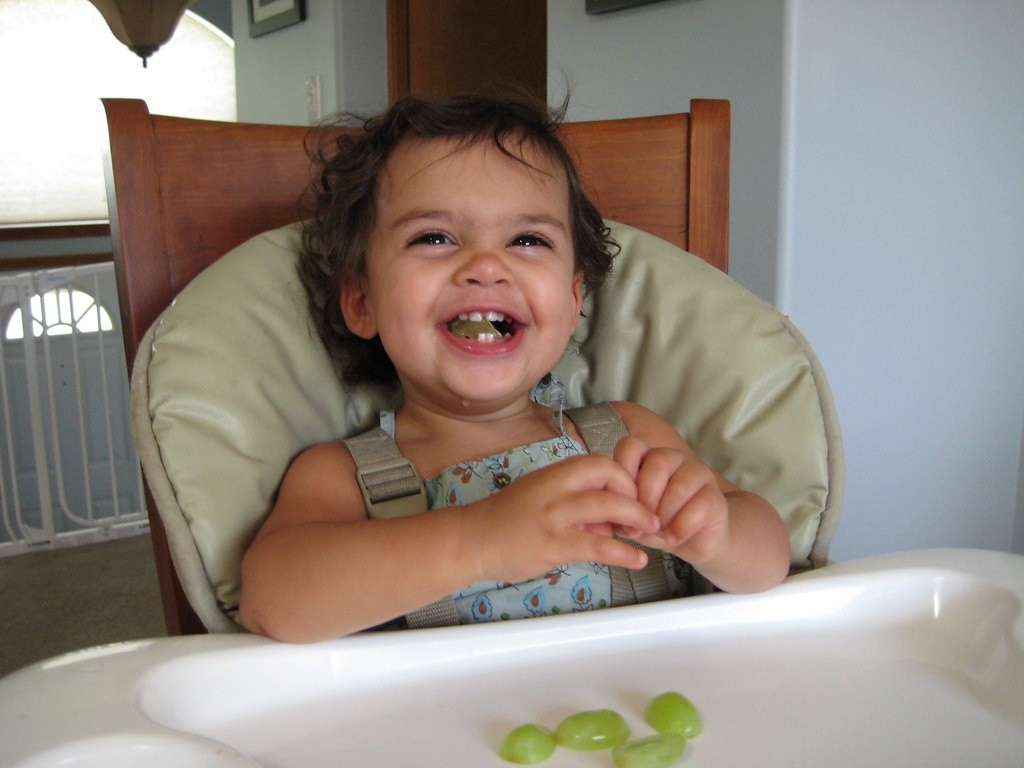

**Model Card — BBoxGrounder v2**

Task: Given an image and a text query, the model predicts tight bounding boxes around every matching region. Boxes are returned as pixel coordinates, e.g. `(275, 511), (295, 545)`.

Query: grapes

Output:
(451, 318), (503, 338)
(499, 690), (704, 768)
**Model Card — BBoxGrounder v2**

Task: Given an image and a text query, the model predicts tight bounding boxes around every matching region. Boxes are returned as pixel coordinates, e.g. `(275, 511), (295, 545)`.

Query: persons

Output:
(240, 96), (792, 643)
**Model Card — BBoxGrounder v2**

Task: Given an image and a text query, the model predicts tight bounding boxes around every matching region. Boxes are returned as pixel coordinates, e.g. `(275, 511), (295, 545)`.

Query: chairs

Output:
(98, 96), (731, 633)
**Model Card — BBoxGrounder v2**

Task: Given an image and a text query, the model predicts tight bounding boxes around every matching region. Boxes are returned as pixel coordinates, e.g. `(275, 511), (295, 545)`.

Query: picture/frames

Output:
(246, 0), (303, 39)
